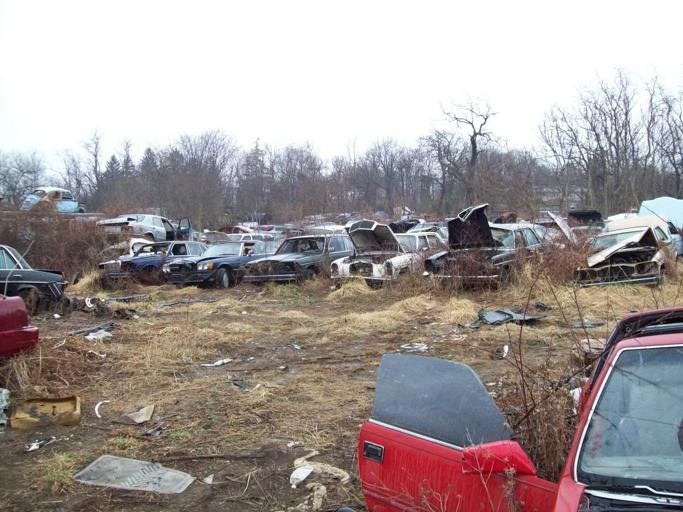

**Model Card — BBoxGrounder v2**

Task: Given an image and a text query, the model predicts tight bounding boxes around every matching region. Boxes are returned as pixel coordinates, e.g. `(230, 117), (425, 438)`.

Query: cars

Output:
(19, 186), (87, 214)
(0, 244), (71, 317)
(359, 308), (683, 512)
(95, 186), (683, 290)
(0, 292), (39, 355)
